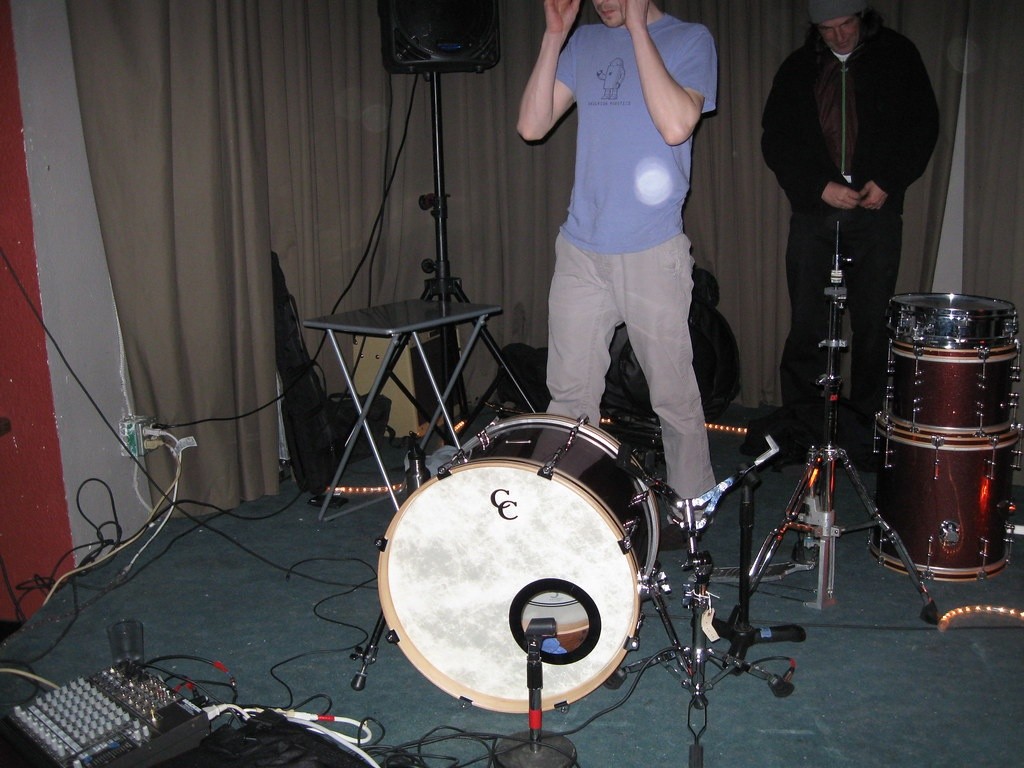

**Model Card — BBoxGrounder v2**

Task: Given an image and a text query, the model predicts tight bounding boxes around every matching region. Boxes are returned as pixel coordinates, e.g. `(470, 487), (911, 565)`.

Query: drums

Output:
(880, 338), (1020, 437)
(376, 413), (660, 714)
(885, 292), (1019, 349)
(867, 412), (1021, 582)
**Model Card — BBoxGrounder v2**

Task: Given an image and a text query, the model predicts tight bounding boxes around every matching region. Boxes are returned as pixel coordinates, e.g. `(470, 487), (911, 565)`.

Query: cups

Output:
(107, 618), (145, 669)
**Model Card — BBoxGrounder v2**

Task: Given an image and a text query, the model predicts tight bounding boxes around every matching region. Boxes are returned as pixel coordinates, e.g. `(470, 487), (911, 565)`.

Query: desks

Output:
(304, 296), (504, 518)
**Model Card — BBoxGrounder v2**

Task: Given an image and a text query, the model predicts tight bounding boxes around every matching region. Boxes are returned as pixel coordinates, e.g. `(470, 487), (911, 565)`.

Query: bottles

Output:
(406, 431), (431, 495)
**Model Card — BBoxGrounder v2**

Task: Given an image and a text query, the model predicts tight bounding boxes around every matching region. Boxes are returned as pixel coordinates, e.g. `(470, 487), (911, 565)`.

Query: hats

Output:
(807, 0), (866, 24)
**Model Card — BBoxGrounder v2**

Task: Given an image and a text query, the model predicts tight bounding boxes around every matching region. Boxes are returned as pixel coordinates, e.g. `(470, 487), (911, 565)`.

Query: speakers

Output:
(376, 0), (500, 75)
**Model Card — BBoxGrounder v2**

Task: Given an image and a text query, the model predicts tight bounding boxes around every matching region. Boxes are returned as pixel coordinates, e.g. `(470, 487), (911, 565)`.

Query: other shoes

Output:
(851, 425), (882, 470)
(667, 505), (714, 529)
(773, 442), (808, 472)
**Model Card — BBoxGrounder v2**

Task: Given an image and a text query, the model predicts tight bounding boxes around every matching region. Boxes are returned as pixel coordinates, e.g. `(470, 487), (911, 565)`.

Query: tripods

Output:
(349, 249), (938, 700)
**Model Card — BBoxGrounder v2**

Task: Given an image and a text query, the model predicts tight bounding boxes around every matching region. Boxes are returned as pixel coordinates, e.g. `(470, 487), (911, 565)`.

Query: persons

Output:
(516, 0), (720, 553)
(756, 3), (940, 473)
